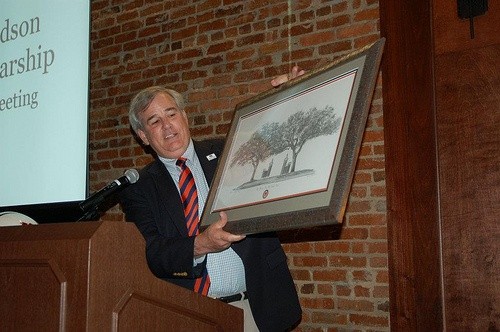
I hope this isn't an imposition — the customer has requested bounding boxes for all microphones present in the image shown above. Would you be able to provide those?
[79,169,140,212]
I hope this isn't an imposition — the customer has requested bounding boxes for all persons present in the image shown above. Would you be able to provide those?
[118,66,306,332]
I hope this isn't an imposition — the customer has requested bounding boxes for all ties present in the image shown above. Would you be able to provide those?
[175,157,211,297]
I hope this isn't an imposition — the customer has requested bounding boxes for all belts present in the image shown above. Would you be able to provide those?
[214,292,249,304]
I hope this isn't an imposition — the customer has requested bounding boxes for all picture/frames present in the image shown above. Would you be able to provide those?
[198,37,386,236]
